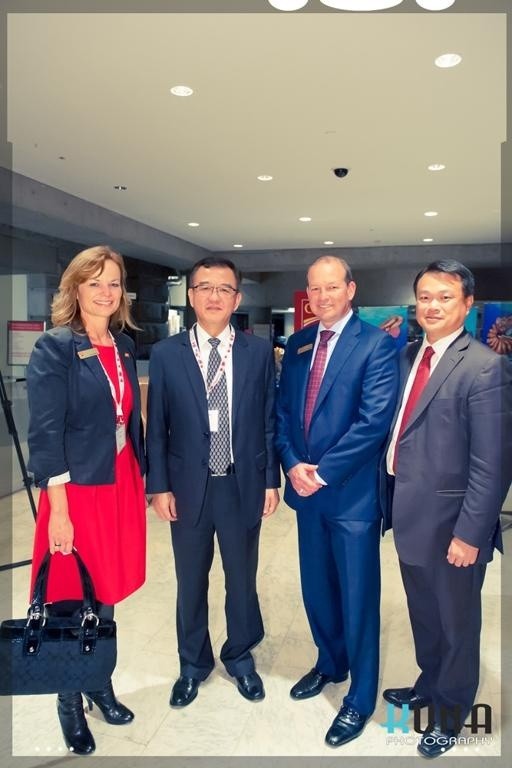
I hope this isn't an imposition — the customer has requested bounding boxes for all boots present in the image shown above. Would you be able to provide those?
[83,601,134,725]
[44,601,95,755]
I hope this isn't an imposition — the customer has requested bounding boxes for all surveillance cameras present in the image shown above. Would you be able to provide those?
[336,168,348,177]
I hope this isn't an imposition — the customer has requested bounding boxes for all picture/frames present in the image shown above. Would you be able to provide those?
[7,320,45,366]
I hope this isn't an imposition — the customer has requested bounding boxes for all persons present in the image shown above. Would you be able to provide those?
[379,259,512,758]
[25,246,148,755]
[146,257,281,706]
[275,255,401,747]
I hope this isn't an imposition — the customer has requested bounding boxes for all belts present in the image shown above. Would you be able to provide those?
[209,463,236,477]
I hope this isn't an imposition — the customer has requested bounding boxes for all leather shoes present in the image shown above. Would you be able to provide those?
[169,666,214,707]
[417,722,458,758]
[383,687,430,711]
[325,703,367,747]
[290,667,348,699]
[236,671,265,701]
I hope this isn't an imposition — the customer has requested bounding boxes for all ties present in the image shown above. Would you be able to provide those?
[303,330,336,438]
[206,337,232,474]
[393,345,436,473]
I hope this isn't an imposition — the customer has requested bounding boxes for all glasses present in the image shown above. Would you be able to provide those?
[192,284,241,297]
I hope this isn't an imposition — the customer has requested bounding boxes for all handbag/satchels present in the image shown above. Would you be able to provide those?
[0,609,118,697]
